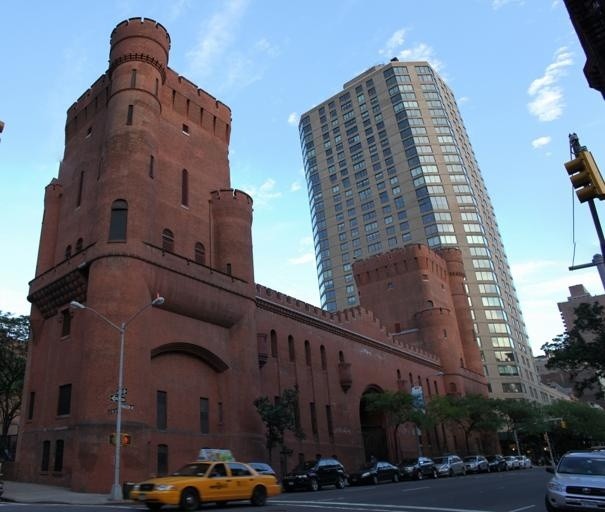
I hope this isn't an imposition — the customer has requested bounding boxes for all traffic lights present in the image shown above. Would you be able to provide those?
[564,153,605,204]
[105,430,133,448]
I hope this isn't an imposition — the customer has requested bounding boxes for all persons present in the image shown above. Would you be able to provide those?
[370,455,376,464]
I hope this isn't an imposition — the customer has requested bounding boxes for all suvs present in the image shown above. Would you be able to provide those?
[544,449,605,512]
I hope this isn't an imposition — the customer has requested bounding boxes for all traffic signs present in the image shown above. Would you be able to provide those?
[104,386,135,417]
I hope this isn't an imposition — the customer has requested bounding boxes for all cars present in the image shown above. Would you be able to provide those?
[400,456,439,480]
[281,455,349,491]
[130,449,281,506]
[243,460,279,484]
[432,455,466,478]
[346,462,401,485]
[464,453,533,473]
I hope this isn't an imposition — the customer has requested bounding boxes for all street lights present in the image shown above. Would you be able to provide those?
[70,295,171,502]
